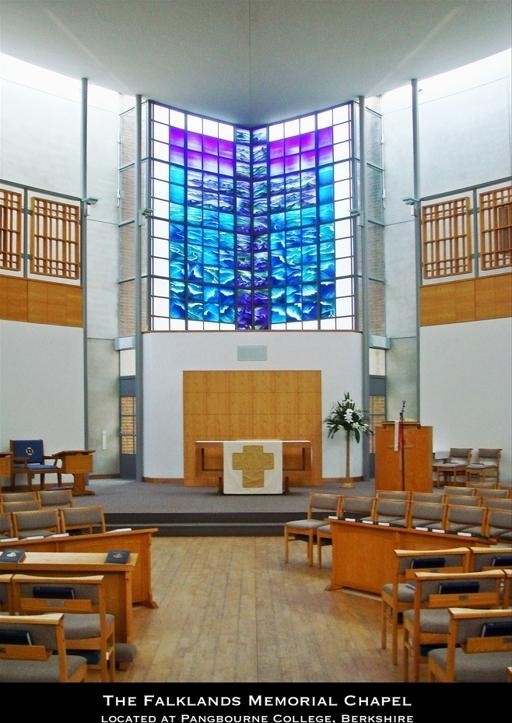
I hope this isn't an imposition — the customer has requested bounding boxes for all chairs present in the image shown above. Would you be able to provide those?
[10,440,62,492]
[436,448,473,488]
[432,452,449,487]
[1,450,159,683]
[462,448,502,489]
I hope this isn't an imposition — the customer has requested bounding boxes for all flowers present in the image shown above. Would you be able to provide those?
[323,392,375,443]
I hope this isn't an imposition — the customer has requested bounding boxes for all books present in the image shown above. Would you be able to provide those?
[0,549,26,564]
[410,556,447,569]
[0,628,33,645]
[490,555,511,566]
[32,585,76,599]
[0,531,70,543]
[437,581,480,594]
[104,549,130,564]
[328,514,489,540]
[105,528,133,533]
[480,621,512,636]
